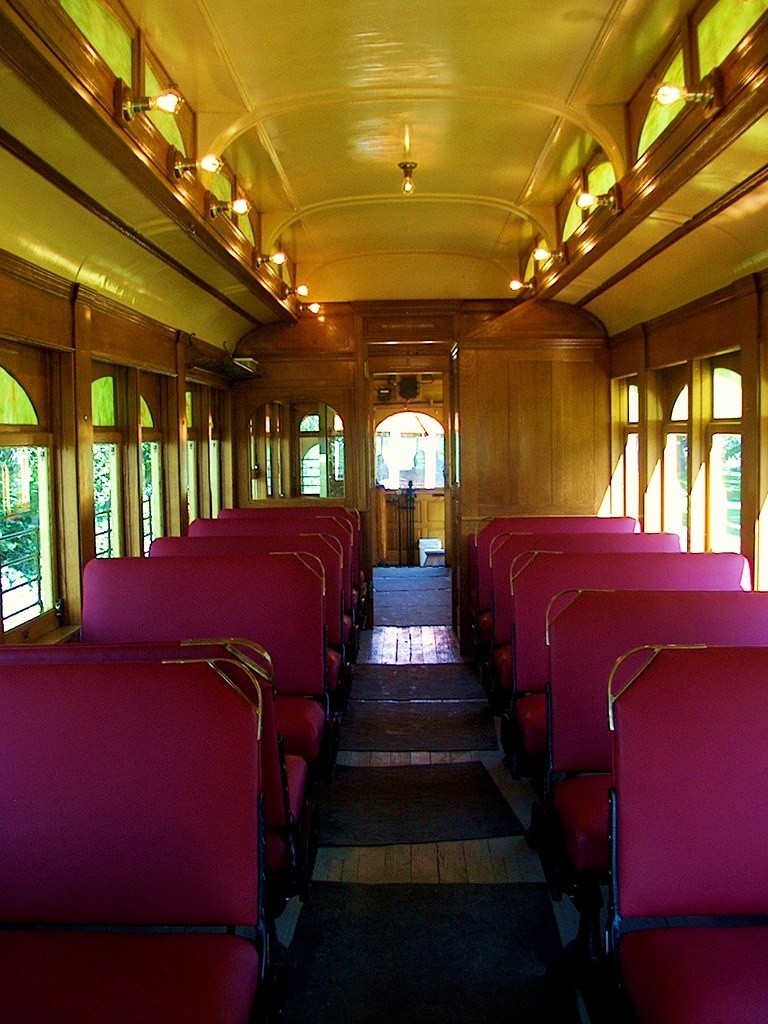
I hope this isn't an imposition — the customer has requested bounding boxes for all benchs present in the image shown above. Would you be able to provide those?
[0,507,768,1024]
